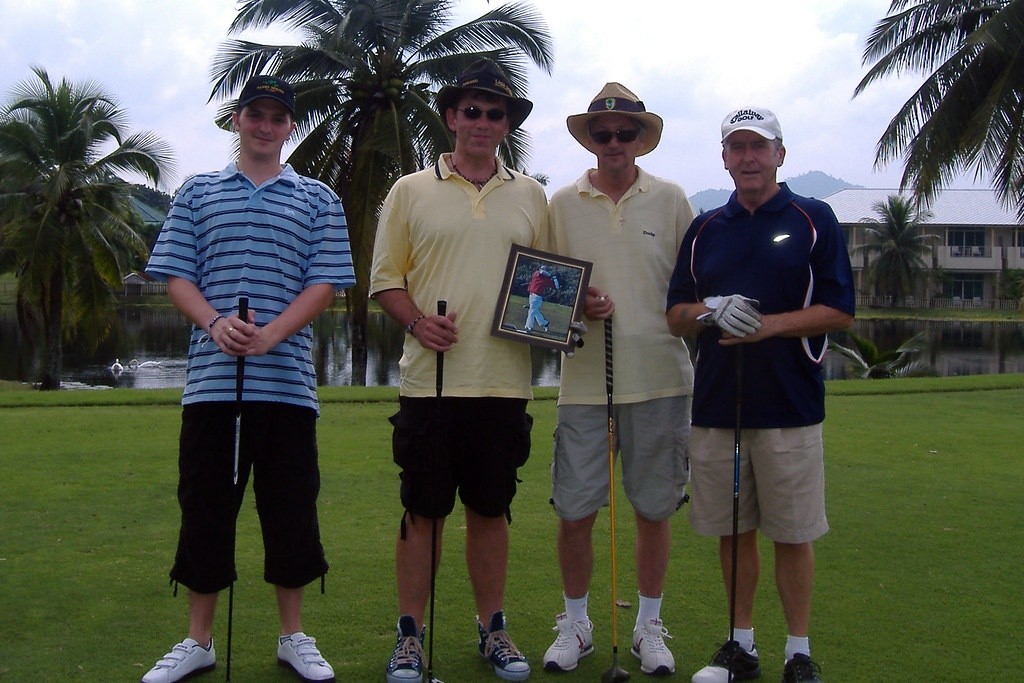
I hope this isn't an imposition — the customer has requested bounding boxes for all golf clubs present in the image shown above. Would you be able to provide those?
[422,300,450,683]
[223,293,250,682]
[601,292,632,682]
[728,340,746,683]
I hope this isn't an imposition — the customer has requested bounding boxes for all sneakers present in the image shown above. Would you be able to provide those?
[385,615,428,683]
[543,612,594,671]
[476,611,530,681]
[691,640,761,683]
[782,653,822,683]
[630,616,675,674]
[278,631,336,683]
[141,638,215,683]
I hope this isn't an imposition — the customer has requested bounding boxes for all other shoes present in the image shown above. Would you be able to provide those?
[543,321,549,332]
[524,326,533,330]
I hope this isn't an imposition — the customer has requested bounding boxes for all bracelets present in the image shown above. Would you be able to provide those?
[208,314,227,339]
[406,314,425,336]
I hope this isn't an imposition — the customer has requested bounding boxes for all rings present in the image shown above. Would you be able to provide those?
[600,296,605,302]
[228,328,236,337]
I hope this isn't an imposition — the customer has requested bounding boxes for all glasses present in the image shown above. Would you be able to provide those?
[453,106,509,120]
[588,127,641,144]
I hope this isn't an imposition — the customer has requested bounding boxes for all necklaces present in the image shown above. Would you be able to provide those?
[449,154,498,189]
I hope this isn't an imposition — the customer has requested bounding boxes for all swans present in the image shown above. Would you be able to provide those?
[129,359,161,369]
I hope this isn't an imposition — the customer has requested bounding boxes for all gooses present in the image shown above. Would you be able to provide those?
[112,358,123,371]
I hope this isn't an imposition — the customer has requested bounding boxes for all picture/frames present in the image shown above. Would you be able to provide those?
[489,244,594,353]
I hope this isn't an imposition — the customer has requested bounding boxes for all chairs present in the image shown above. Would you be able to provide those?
[952,246,961,256]
[973,297,981,308]
[906,295,914,306]
[972,247,981,257]
[953,296,961,307]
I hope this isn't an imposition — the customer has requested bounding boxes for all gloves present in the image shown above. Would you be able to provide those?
[696,294,762,338]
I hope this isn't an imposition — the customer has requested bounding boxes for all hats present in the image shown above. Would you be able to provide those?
[436,59,533,134]
[237,76,295,118]
[721,107,783,147]
[567,83,662,156]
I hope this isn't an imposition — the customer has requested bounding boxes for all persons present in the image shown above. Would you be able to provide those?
[525,265,560,331]
[665,105,856,683]
[369,57,549,683]
[542,80,697,673]
[142,75,357,683]
